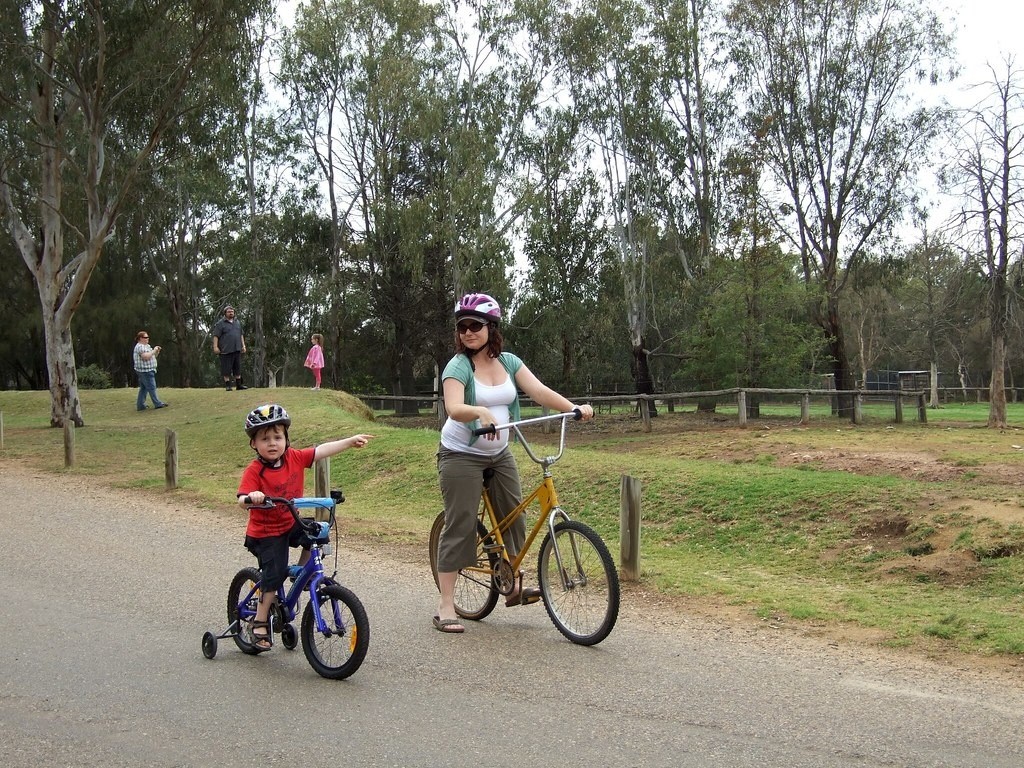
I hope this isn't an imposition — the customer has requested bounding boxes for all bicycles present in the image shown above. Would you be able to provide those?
[428,406,621,646]
[200,490,370,680]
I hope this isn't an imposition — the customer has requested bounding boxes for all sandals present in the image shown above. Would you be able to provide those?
[247,615,272,654]
[290,565,318,591]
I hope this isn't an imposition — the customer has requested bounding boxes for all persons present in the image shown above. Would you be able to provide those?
[237,402,375,651]
[304,334,325,391]
[431,292,592,632]
[133,331,168,411]
[212,307,251,391]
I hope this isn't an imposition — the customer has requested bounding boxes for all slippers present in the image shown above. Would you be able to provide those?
[433,616,465,632]
[505,587,542,607]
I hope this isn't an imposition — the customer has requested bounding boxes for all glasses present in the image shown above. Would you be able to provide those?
[457,321,490,334]
[142,336,150,339]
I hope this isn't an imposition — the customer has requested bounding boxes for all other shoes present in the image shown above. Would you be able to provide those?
[155,403,168,410]
[311,387,320,391]
[236,384,248,390]
[137,406,149,412]
[226,386,232,391]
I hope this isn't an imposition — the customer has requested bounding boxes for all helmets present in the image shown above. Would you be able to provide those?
[245,404,291,438]
[454,293,501,326]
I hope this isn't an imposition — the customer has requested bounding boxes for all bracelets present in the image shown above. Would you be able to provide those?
[572,404,578,411]
[242,346,246,348]
